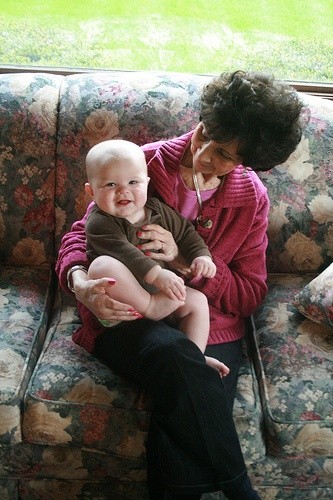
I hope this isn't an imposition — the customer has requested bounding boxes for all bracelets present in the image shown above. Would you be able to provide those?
[66,265,88,292]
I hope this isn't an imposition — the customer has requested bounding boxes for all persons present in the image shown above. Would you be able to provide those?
[56,68,311,500]
[84,139,231,381]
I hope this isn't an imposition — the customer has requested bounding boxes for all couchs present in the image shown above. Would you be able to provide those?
[0,72,332,499]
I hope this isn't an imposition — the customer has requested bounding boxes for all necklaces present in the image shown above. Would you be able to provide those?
[192,168,225,224]
